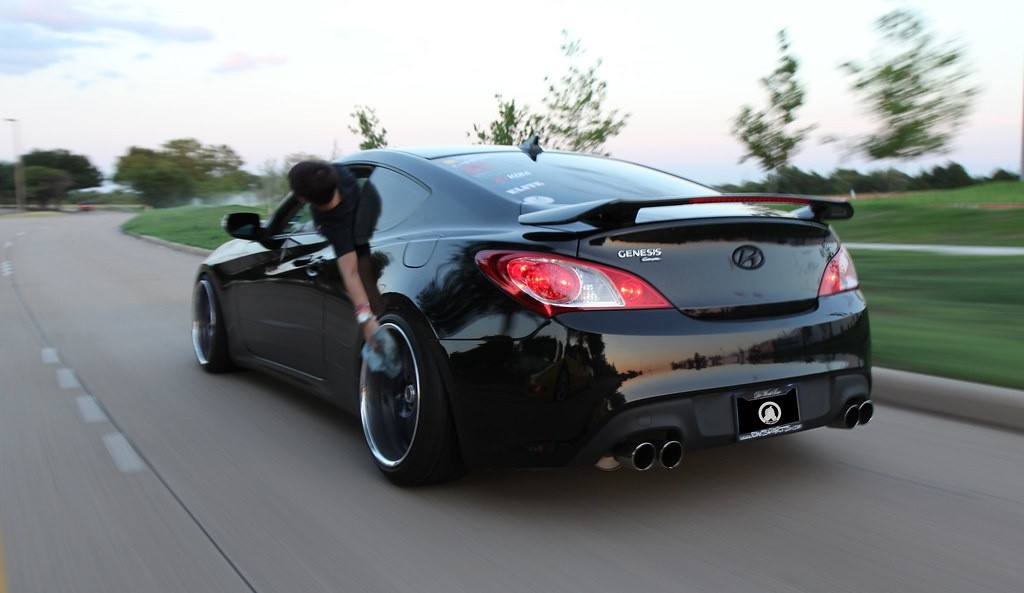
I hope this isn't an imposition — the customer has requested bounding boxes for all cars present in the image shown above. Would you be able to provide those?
[191,134,874,486]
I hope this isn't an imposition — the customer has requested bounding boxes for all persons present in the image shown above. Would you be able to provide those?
[290,159,390,358]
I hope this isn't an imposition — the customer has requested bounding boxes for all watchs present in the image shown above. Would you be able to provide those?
[357,312,374,324]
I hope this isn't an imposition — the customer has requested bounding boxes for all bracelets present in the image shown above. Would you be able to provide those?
[352,304,370,316]
